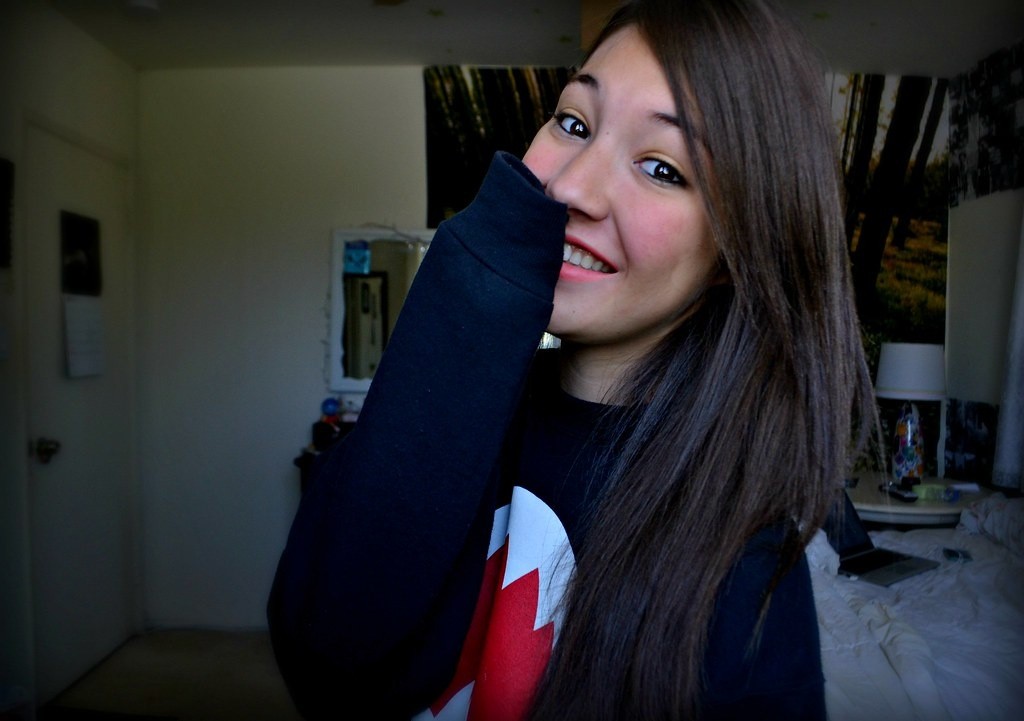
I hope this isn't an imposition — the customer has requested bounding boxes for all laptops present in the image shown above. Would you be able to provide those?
[820,487,941,588]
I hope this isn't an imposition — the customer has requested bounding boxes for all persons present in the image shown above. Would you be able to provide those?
[266,0,888,720]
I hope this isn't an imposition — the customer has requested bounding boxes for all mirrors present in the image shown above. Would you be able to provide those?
[346,238,429,379]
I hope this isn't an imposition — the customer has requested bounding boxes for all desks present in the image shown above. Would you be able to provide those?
[848,476,987,530]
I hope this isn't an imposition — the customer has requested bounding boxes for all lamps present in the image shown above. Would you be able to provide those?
[875,342,949,488]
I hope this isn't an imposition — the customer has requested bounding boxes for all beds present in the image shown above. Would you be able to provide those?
[800,500,1024,721]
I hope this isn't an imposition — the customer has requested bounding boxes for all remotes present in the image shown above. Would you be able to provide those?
[879,483,919,502]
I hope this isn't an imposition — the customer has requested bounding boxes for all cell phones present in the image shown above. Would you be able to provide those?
[944,548,972,561]
